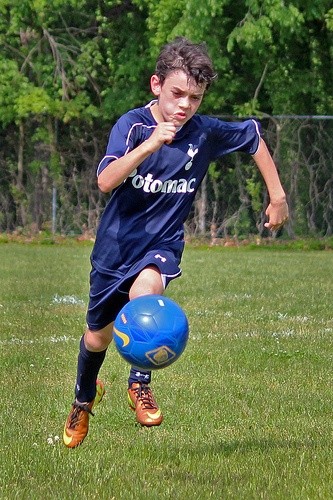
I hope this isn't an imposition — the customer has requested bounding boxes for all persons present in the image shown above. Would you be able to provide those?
[61,43,289,449]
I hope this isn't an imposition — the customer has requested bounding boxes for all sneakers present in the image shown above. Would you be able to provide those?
[126,382,163,427]
[63,380,105,449]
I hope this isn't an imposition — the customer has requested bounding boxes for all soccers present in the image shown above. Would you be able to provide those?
[112,294,189,369]
